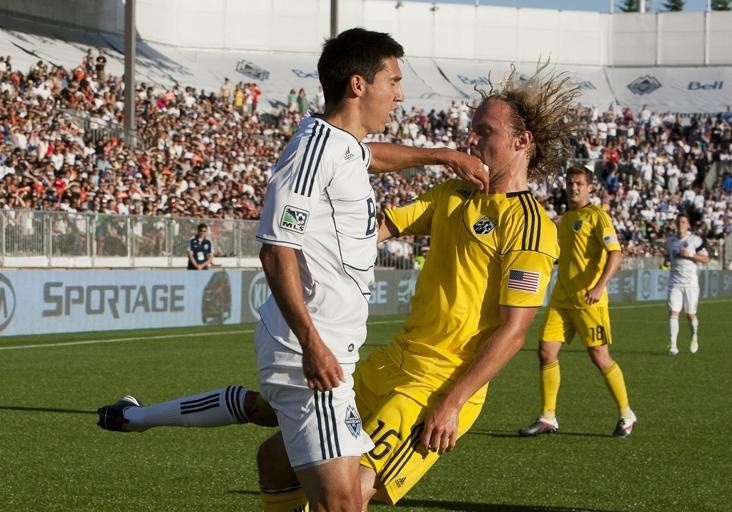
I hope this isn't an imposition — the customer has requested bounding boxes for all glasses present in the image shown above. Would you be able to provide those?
[199,230,207,232]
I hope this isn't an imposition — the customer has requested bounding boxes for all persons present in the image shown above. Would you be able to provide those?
[661,214,709,353]
[518,164,638,438]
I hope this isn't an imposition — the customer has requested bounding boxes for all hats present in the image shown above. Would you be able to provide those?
[0,49,109,77]
[0,78,260,216]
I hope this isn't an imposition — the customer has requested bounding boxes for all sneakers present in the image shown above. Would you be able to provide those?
[97,395,144,432]
[519,416,558,436]
[613,411,637,438]
[667,348,678,355]
[689,335,698,353]
